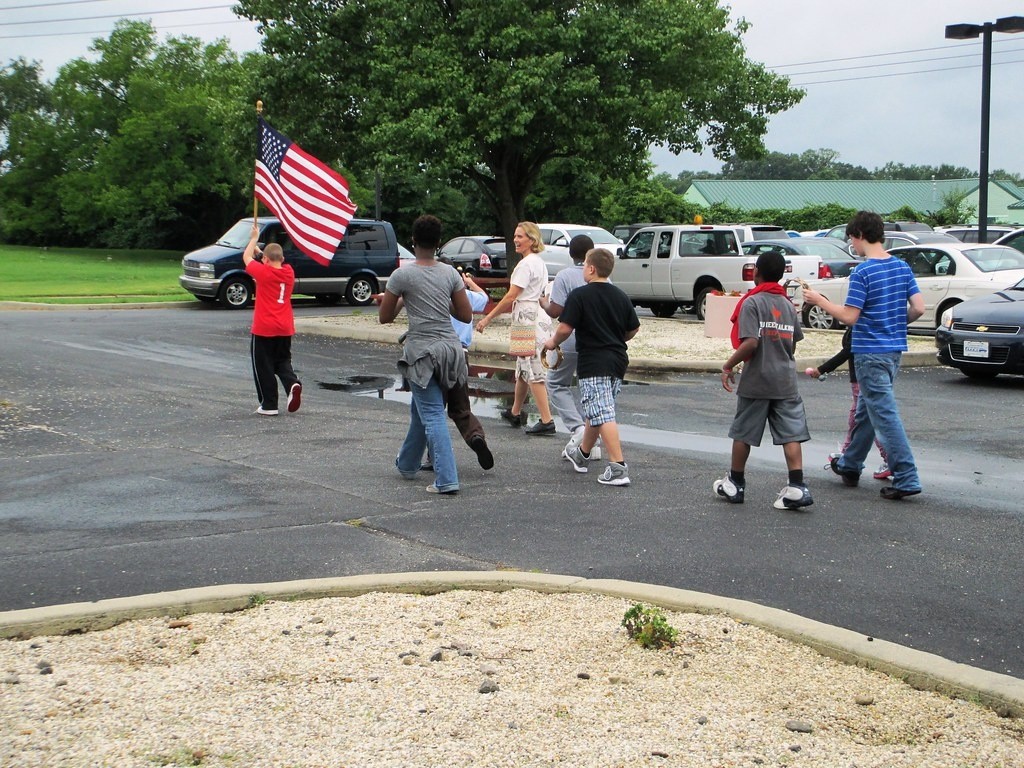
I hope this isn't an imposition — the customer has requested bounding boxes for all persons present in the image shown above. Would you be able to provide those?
[538,234,613,461]
[805,326,892,479]
[713,251,814,510]
[243,226,302,415]
[544,249,640,486]
[370,257,494,471]
[379,216,473,494]
[475,222,558,434]
[802,211,926,500]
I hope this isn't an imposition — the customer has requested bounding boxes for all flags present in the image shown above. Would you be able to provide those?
[254,114,358,266]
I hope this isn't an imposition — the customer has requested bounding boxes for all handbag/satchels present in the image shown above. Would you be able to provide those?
[508,326,536,356]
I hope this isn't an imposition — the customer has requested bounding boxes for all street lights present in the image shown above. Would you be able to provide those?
[945,16,1024,243]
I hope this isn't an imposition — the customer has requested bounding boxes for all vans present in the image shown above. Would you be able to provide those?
[179,216,401,309]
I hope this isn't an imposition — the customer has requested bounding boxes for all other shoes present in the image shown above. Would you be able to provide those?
[397,452,400,458]
[420,463,434,470]
[426,485,459,493]
[471,436,494,470]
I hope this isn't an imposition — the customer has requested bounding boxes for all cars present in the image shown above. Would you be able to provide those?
[399,242,416,274]
[533,223,1024,383]
[435,237,507,277]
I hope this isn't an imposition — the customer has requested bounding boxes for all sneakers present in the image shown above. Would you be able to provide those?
[831,457,860,487]
[873,464,891,479]
[880,487,922,499]
[713,471,747,504]
[500,409,522,428]
[287,383,302,412]
[565,445,591,472]
[828,453,844,462]
[256,406,278,416]
[588,446,602,460]
[524,419,557,436]
[561,424,585,460]
[597,461,630,485]
[773,479,814,510]
